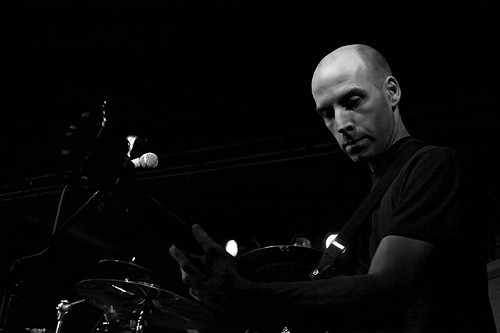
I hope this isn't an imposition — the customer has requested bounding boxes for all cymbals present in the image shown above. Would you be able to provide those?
[75,278,215,331]
[97,258,154,281]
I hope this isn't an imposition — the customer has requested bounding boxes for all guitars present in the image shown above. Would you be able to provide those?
[1,96,110,327]
[65,99,374,283]
[1,150,160,202]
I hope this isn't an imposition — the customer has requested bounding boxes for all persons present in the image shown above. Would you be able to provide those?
[168,43,497,333]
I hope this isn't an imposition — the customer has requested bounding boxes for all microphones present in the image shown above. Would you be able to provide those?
[80,152,159,182]
